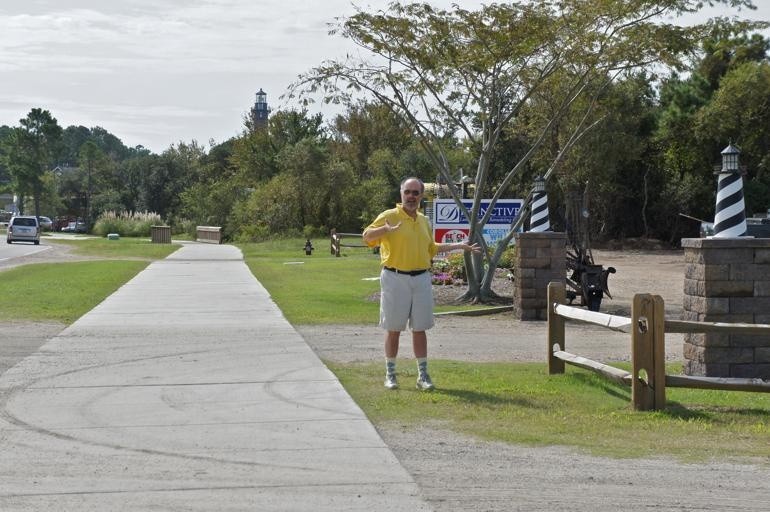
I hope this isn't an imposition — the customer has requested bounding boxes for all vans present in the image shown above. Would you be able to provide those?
[4,216,40,244]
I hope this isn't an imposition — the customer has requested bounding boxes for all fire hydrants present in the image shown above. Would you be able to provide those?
[301,239,315,256]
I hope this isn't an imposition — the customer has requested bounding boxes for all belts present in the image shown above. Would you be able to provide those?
[384,266,426,276]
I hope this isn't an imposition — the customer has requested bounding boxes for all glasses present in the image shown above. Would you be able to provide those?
[404,190,419,195]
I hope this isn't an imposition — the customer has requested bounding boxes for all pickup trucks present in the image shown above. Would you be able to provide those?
[699,220,770,239]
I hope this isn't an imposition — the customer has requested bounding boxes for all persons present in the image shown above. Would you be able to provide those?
[362,176,483,393]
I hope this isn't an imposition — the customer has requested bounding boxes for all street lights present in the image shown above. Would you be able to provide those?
[461,175,471,198]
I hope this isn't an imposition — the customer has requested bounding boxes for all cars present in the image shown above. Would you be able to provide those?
[0,213,85,233]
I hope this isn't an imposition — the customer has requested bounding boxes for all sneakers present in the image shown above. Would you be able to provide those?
[385,374,398,388]
[416,375,433,390]
